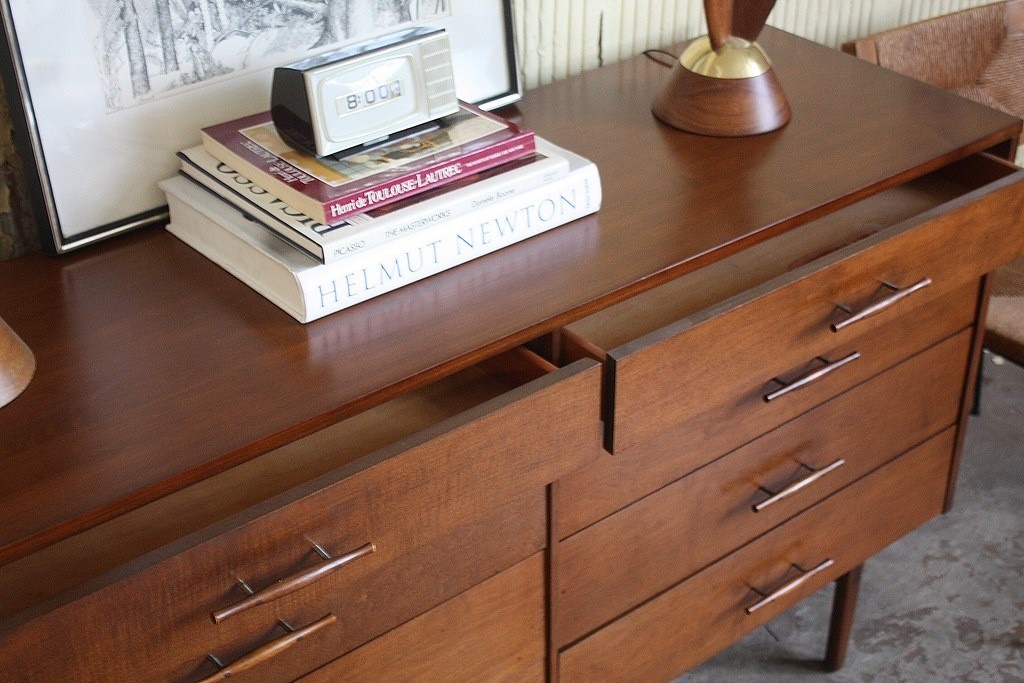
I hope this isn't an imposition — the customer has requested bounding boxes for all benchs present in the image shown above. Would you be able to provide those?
[842,0,1024,415]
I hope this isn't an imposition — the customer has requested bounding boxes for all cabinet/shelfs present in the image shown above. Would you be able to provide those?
[0,22,1023,683]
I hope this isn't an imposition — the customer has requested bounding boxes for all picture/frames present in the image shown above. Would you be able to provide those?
[0,0,523,253]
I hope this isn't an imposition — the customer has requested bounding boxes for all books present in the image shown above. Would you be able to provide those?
[152,99,602,324]
